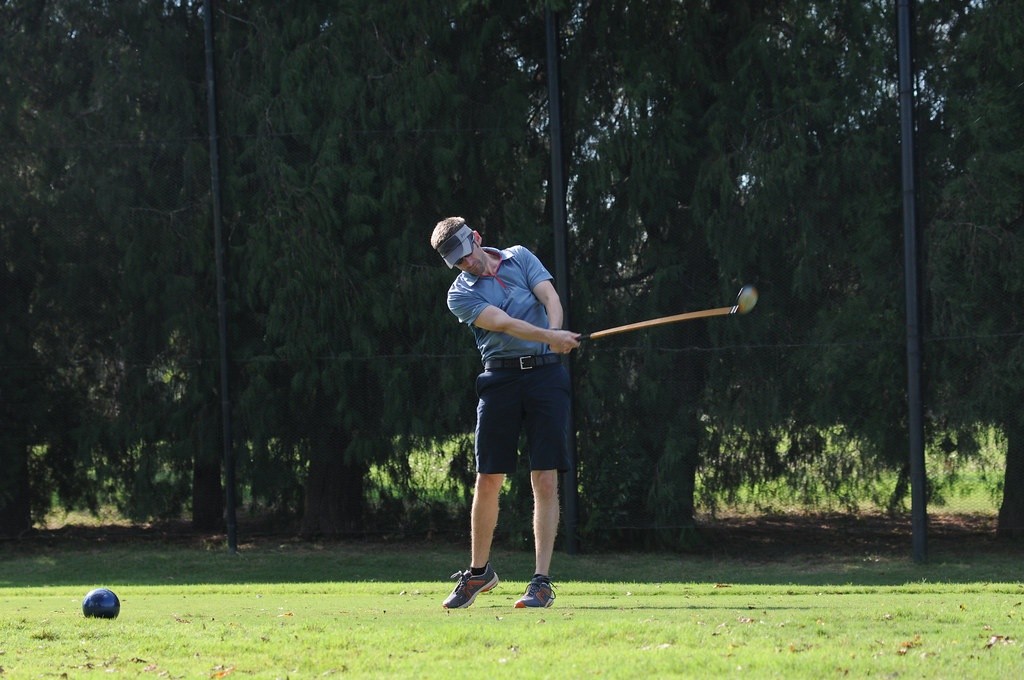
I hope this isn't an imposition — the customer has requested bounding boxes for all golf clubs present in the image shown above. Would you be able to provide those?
[573,283,760,341]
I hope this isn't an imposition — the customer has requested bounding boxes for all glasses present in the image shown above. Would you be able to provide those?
[456,236,475,264]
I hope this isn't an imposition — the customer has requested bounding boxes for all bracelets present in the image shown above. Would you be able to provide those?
[553,329,560,330]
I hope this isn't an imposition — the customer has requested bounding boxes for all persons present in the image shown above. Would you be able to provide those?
[431,217,581,612]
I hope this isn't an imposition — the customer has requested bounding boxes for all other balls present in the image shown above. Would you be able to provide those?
[84,588,121,620]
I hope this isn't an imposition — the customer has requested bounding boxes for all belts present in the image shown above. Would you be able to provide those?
[485,354,561,370]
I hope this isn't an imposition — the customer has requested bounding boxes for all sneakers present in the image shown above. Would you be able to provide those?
[515,577,557,608]
[443,562,499,609]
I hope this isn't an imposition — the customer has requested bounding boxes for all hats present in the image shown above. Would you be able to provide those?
[437,224,474,270]
